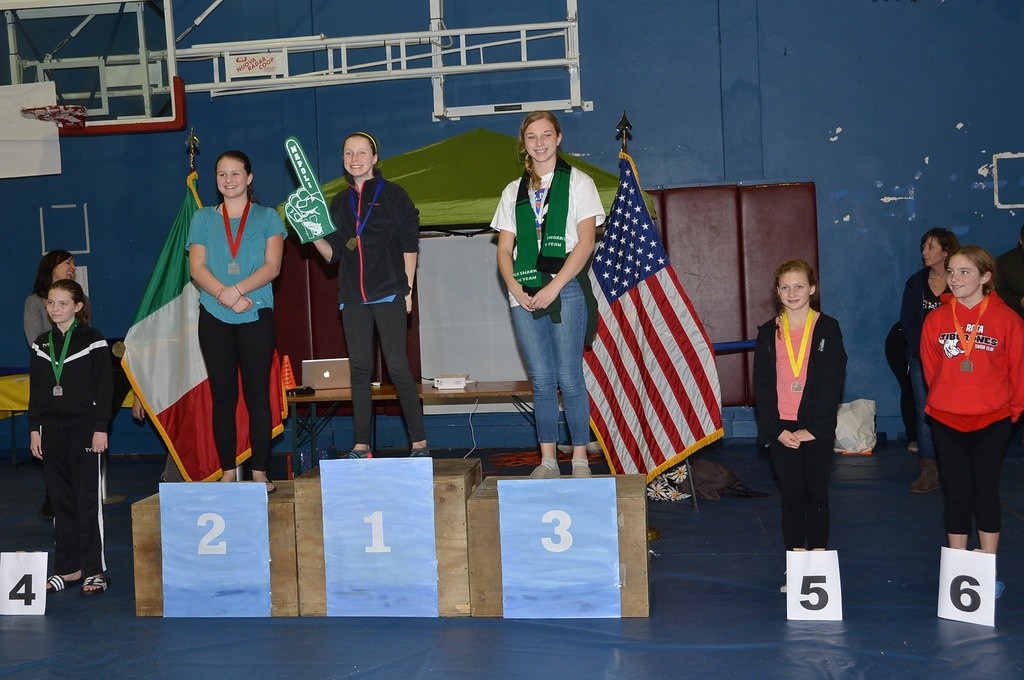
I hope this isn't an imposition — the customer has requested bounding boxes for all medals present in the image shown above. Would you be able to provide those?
[346,237,357,252]
[227,260,240,274]
[53,382,63,396]
[790,379,802,392]
[960,358,973,371]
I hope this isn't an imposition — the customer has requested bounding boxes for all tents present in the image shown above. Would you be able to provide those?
[279,125,700,505]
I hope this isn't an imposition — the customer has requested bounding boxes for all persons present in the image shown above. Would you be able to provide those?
[752,259,848,594]
[886,322,919,455]
[921,244,1024,600]
[22,250,90,347]
[29,279,114,595]
[902,228,959,496]
[312,132,431,459]
[131,393,183,482]
[184,149,290,494]
[994,226,1024,319]
[488,111,606,477]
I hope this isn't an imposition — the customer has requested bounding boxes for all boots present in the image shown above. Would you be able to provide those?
[908,459,941,492]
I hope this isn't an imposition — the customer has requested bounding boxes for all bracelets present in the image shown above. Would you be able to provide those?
[234,284,244,297]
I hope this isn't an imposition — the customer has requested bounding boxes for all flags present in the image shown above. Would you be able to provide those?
[582,149,725,484]
[119,171,288,482]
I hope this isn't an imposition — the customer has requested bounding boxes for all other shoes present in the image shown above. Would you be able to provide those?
[410,448,430,457]
[344,449,372,458]
[995,580,1004,599]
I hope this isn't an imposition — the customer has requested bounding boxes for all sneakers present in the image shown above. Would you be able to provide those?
[571,461,592,478]
[527,465,561,479]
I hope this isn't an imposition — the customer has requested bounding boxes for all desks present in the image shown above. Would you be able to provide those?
[282,376,565,478]
[0,369,141,470]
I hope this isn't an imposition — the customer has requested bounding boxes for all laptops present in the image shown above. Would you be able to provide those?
[301,358,351,389]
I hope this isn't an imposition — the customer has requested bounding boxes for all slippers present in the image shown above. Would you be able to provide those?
[253,476,277,493]
[47,574,83,593]
[81,577,111,595]
